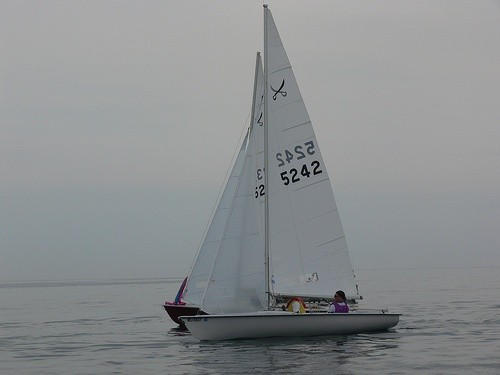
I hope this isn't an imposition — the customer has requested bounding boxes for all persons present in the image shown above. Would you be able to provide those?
[287,296,306,313]
[328,291,350,313]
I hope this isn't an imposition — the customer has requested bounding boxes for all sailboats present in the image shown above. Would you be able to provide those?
[162,51,264,326]
[179,4,403,341]
